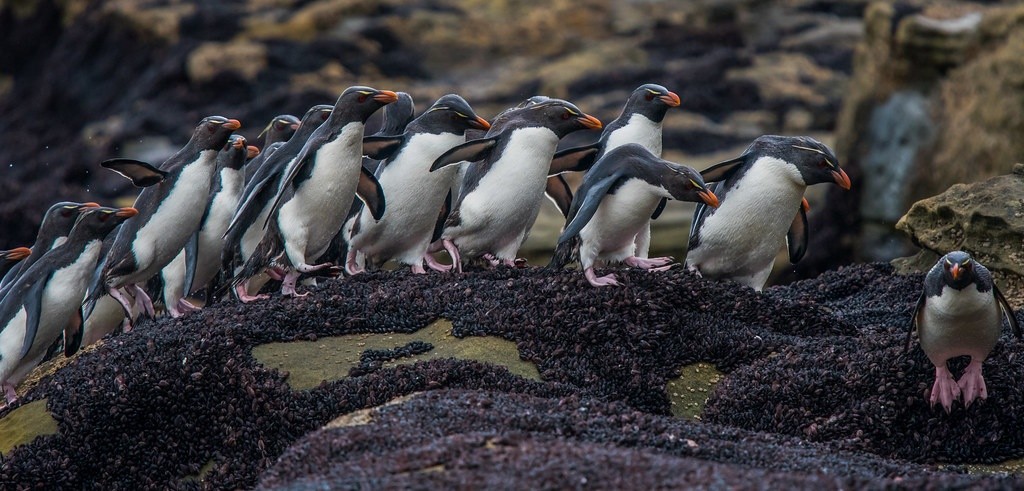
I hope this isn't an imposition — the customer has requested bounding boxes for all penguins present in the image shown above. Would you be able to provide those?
[0,84,850,410]
[904,251,1021,415]
